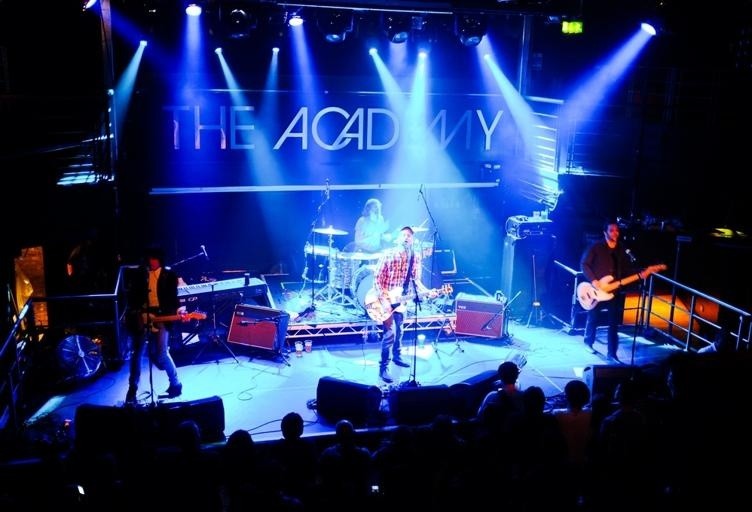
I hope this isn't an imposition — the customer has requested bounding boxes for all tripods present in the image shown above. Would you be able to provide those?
[265,320,291,368]
[526,255,556,330]
[407,227,442,313]
[291,218,342,324]
[191,286,240,363]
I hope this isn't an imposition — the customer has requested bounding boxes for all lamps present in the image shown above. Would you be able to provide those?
[83,0,670,42]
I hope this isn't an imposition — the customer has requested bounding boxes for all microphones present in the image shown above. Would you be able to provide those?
[200,244,208,258]
[325,178,330,200]
[417,183,423,200]
[625,248,637,261]
[405,243,416,256]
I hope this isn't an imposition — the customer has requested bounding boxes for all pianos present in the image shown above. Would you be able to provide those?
[178,278,267,307]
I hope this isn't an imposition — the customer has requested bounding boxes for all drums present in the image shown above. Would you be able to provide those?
[302,245,338,284]
[351,266,377,309]
[330,251,372,288]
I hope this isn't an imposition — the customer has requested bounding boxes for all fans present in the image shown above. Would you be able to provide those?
[57,335,104,380]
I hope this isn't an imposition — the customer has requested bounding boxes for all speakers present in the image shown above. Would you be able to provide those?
[500,233,555,315]
[666,343,732,401]
[388,385,450,424]
[227,304,290,353]
[582,363,640,411]
[74,403,153,443]
[157,396,226,443]
[316,376,382,427]
[450,369,498,415]
[454,292,506,339]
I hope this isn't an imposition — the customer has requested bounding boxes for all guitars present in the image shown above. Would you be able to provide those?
[577,264,667,310]
[365,284,454,322]
[126,313,206,336]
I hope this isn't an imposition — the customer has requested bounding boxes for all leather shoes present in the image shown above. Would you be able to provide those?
[586,342,620,364]
[380,366,392,382]
[159,388,180,398]
[395,360,410,367]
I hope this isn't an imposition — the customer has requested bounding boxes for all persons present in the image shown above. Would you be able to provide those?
[373,225,438,383]
[124,248,189,406]
[354,198,392,253]
[582,219,650,367]
[1,347,733,511]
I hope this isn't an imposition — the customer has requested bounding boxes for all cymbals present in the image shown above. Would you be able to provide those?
[312,229,348,235]
[410,227,429,232]
